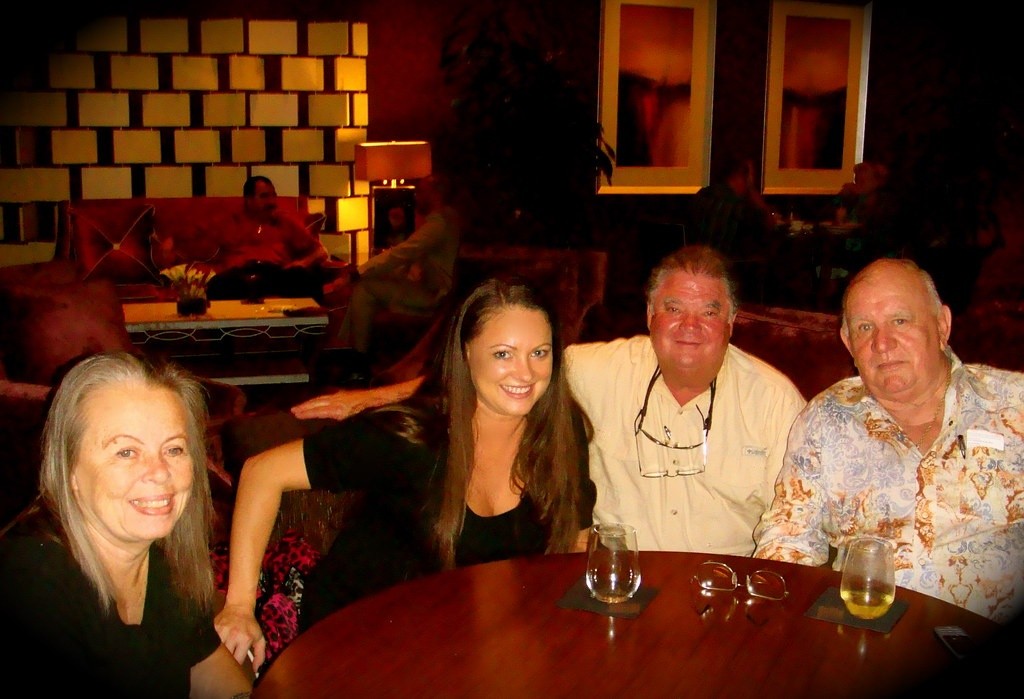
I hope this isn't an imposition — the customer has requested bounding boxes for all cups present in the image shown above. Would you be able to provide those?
[790,221,803,232]
[839,539,895,620]
[834,208,847,226]
[587,524,640,603]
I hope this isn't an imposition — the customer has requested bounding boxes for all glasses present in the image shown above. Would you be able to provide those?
[637,426,707,450]
[635,405,708,478]
[689,562,787,627]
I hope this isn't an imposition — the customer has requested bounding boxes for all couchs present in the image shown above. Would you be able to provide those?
[53,195,342,299]
[729,298,861,404]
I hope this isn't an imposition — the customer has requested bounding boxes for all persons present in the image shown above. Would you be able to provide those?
[815,163,892,301]
[752,257,1023,635]
[0,350,253,699]
[323,171,460,387]
[962,185,1024,318]
[194,176,329,300]
[291,245,807,558]
[214,275,598,687]
[669,150,781,306]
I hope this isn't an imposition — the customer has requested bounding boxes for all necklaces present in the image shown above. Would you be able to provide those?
[916,359,951,450]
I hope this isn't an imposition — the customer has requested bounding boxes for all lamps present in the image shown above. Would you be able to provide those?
[356,138,433,250]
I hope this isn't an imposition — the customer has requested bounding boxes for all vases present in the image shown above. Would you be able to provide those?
[178,295,205,317]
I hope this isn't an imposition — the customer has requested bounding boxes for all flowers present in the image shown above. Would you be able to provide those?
[160,263,216,300]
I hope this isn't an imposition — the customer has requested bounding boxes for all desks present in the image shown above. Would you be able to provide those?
[122,296,328,385]
[250,550,1024,699]
